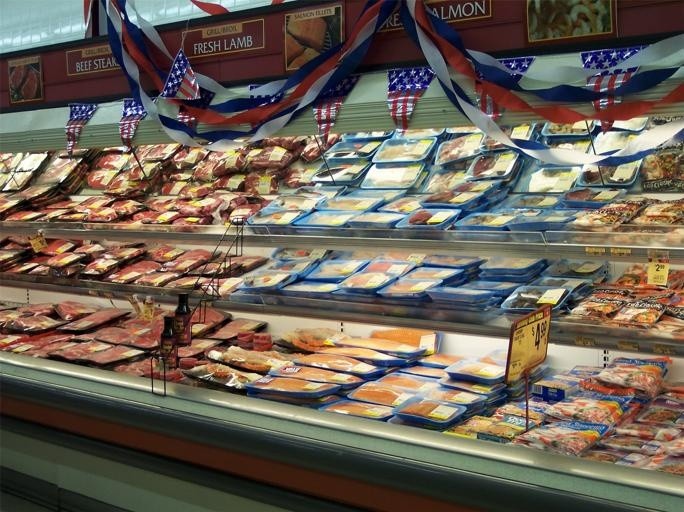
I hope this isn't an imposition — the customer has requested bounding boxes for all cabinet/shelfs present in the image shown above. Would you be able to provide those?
[0,50,684,512]
